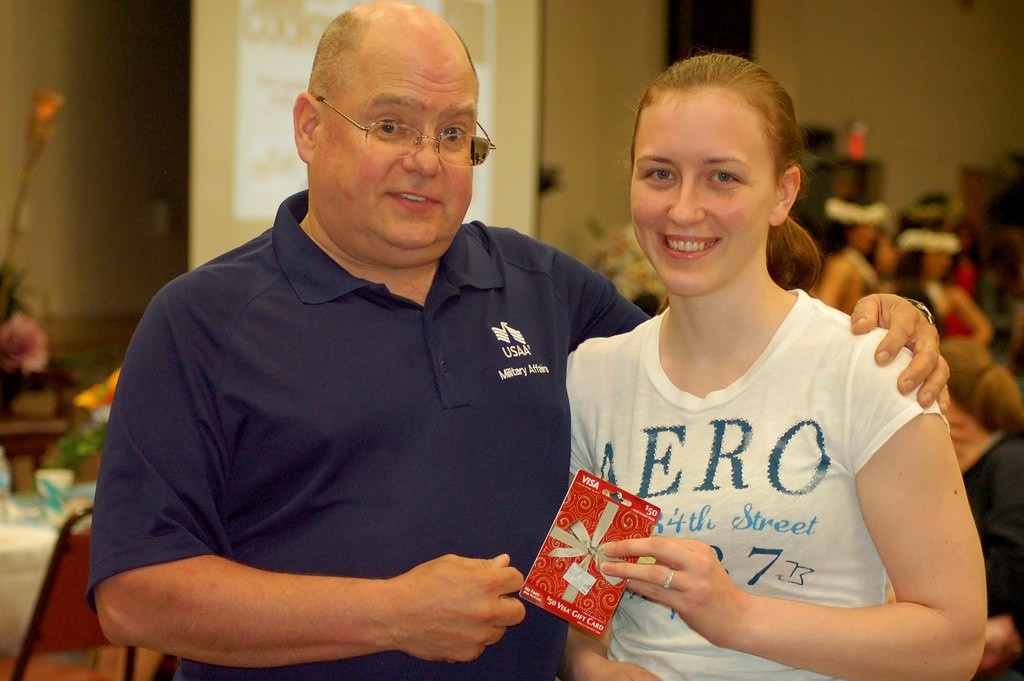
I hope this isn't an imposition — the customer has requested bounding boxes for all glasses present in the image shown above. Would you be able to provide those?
[316,94,496,168]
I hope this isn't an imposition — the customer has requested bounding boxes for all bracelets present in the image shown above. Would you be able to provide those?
[908,298,936,326]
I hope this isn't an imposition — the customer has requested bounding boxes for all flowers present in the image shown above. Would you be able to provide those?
[47,366,120,472]
[0,90,64,418]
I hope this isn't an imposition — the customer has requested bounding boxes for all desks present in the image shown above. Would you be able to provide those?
[1,492,164,680]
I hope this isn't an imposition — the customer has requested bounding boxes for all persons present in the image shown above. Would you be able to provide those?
[565,53,989,681]
[85,0,950,681]
[819,195,1023,680]
[0,272,49,414]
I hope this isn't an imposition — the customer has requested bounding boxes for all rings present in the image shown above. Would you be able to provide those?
[664,567,675,589]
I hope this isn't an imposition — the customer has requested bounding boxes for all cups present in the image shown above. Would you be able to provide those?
[846,121,868,158]
[34,468,75,498]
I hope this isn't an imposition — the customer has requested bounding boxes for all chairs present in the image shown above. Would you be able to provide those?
[9,505,134,681]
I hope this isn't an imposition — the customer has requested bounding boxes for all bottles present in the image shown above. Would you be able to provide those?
[0,447,15,523]
